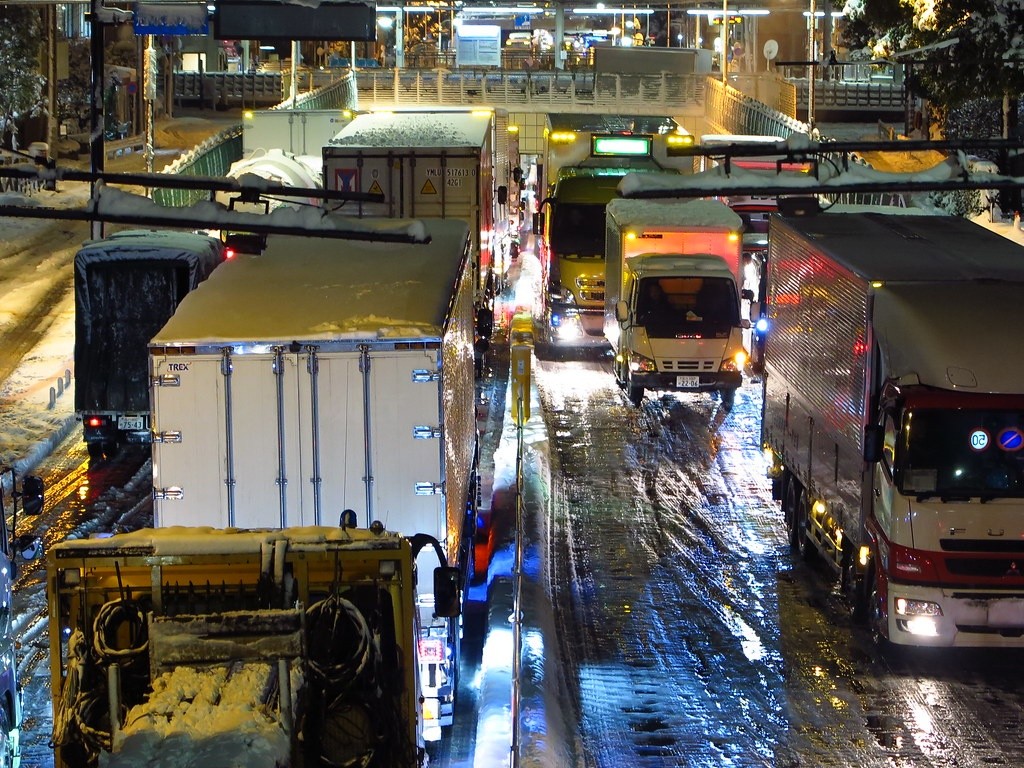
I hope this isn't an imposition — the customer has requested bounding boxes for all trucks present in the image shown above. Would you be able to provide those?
[321,112,526,336]
[147,217,494,741]
[602,198,750,404]
[700,135,817,276]
[0,464,44,768]
[533,113,695,348]
[74,230,228,459]
[760,212,1023,647]
[47,509,460,768]
